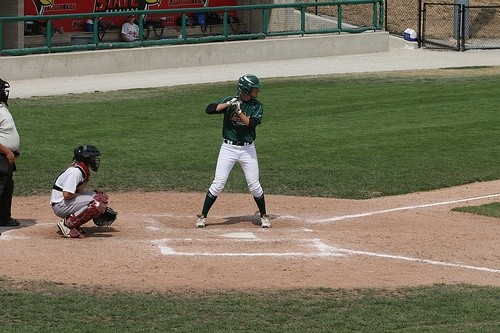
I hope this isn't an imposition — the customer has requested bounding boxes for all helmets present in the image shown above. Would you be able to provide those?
[74,145,100,172]
[0,78,10,105]
[238,75,261,94]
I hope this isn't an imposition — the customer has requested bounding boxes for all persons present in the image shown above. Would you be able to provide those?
[121,15,139,42]
[195,74,271,228]
[0,79,20,227]
[50,144,109,238]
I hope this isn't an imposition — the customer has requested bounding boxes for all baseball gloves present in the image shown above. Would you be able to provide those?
[92,206,118,226]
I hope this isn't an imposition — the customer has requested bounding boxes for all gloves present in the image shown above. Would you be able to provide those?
[227,97,242,116]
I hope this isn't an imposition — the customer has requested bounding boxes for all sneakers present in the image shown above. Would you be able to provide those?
[57,219,86,239]
[259,214,272,228]
[195,214,206,228]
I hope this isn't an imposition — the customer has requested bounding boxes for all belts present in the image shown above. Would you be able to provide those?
[224,139,252,146]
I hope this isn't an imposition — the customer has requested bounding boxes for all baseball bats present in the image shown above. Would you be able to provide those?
[231,92,240,111]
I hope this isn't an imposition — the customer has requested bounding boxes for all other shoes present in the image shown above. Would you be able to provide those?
[0,217,20,226]
